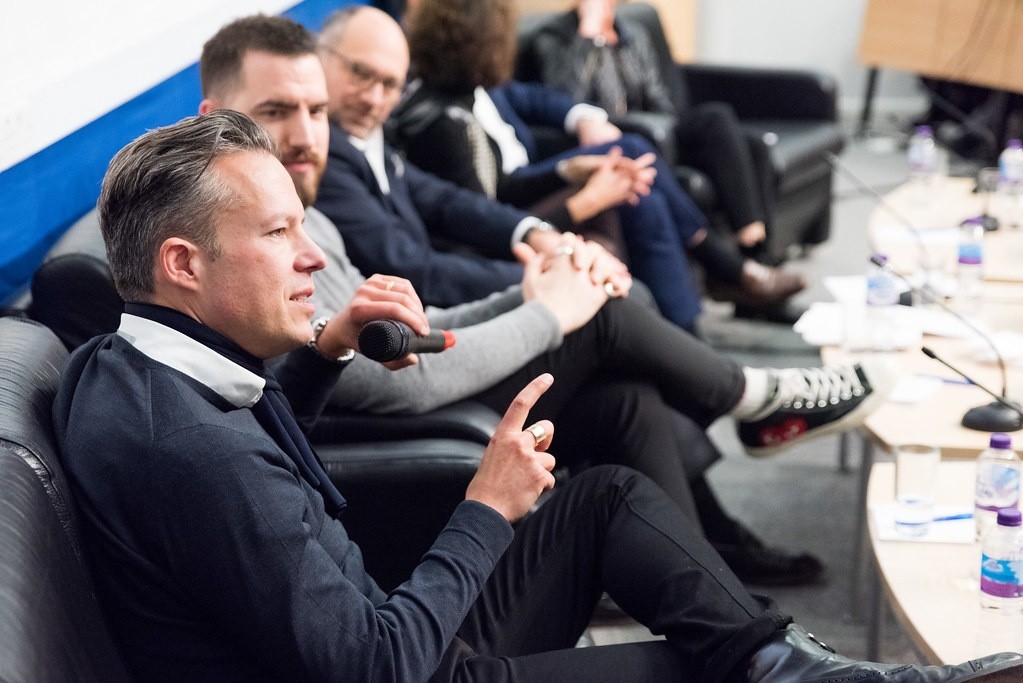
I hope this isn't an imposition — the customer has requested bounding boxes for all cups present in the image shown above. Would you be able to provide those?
[895,444,942,538]
[978,166,1001,231]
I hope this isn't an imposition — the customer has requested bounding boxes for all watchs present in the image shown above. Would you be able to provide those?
[307,317,355,361]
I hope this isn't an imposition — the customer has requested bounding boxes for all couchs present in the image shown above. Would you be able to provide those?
[675,62,846,265]
[0,309,597,683]
[29,206,503,447]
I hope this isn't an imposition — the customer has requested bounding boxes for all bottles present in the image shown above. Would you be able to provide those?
[865,256,896,352]
[998,138,1023,230]
[908,125,938,204]
[981,507,1023,627]
[955,217,984,307]
[974,432,1022,554]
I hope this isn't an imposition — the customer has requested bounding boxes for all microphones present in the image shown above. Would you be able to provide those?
[822,123,1023,432]
[357,317,457,363]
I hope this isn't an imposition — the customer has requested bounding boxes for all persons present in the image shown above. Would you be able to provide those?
[53,108,1022,682]
[312,5,824,581]
[389,0,813,347]
[199,16,884,613]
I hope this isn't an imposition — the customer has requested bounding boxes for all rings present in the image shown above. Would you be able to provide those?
[525,424,546,445]
[386,281,395,292]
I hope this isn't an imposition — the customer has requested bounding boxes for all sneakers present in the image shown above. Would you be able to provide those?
[736,354,896,457]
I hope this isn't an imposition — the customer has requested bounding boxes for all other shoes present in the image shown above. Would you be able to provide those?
[704,261,810,304]
[715,517,823,589]
[736,297,806,324]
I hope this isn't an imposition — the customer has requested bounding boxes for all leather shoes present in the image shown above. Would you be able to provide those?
[740,620,1023,683]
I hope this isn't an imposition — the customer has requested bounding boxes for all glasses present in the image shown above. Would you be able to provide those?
[320,43,405,100]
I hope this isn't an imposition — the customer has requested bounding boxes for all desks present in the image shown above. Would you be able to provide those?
[822,344,1023,624]
[862,459,1023,670]
[798,278,1023,473]
[865,172,1023,281]
[854,0,1023,140]
